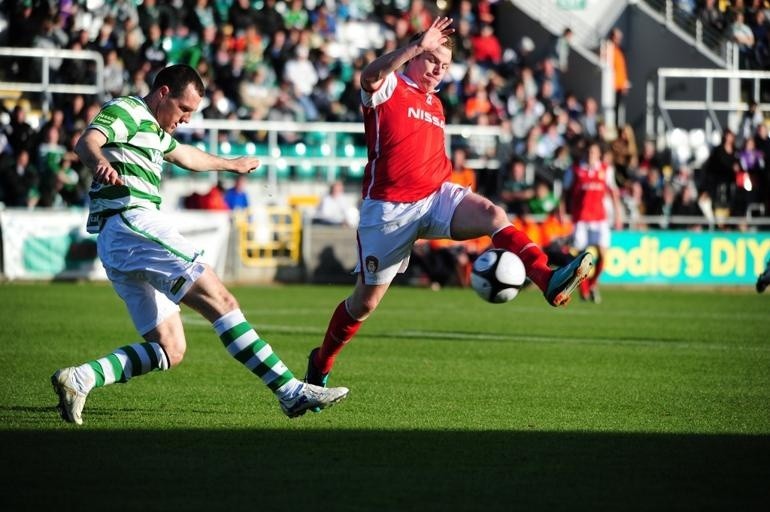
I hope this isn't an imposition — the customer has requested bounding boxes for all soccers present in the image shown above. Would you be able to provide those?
[471,248,526,304]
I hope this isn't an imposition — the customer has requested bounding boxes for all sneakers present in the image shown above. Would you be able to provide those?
[545,249,593,310]
[278,380,350,419]
[303,346,332,414]
[51,365,87,425]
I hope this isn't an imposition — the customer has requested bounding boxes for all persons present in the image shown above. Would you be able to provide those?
[50,64,349,426]
[301,14,594,413]
